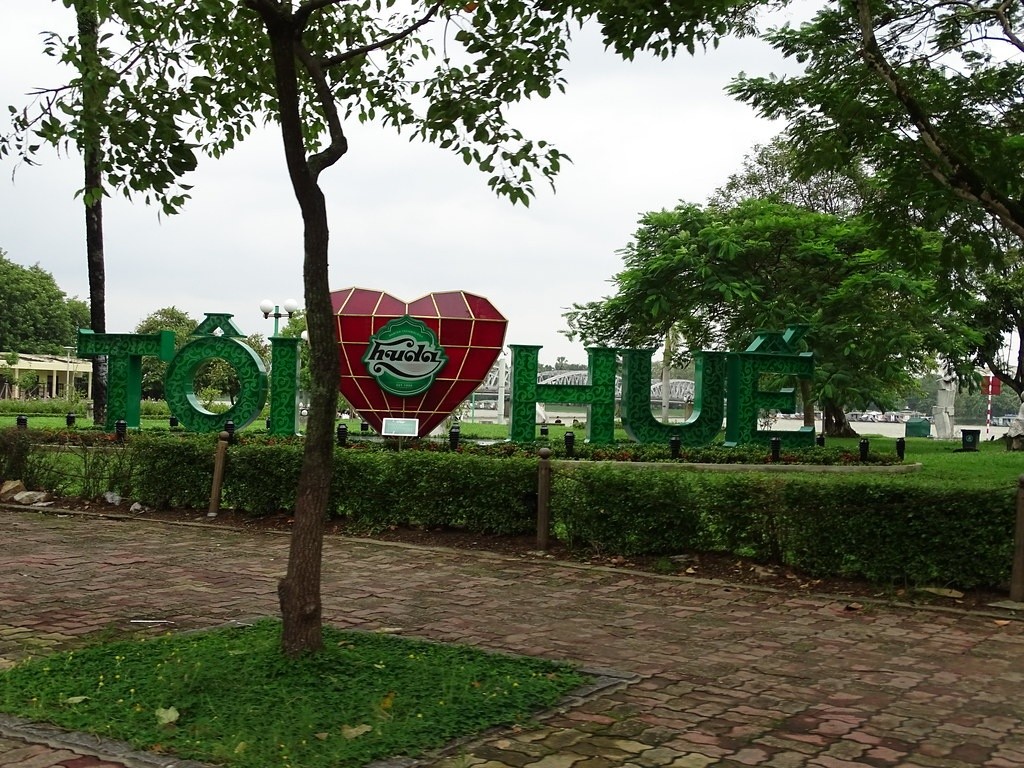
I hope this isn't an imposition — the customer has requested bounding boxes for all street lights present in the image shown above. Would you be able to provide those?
[259,299,298,336]
[63,346,75,402]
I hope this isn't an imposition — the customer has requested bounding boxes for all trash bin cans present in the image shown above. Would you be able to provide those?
[905,417,931,437]
[961,429,981,450]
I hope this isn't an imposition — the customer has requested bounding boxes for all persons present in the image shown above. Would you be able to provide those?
[573,417,578,423]
[555,416,561,424]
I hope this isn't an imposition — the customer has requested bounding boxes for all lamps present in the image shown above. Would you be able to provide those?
[337,423,349,441]
[671,434,681,452]
[361,422,369,430]
[816,434,826,445]
[225,419,235,435]
[16,413,29,427]
[66,410,75,425]
[859,437,870,452]
[266,416,271,429]
[449,421,461,442]
[169,414,179,427]
[541,425,549,435]
[770,436,781,451]
[114,418,127,436]
[896,438,905,451]
[564,430,574,445]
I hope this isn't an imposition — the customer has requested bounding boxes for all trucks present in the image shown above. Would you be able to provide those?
[769,409,825,421]
[845,410,935,424]
[990,417,1016,426]
[459,399,497,410]
